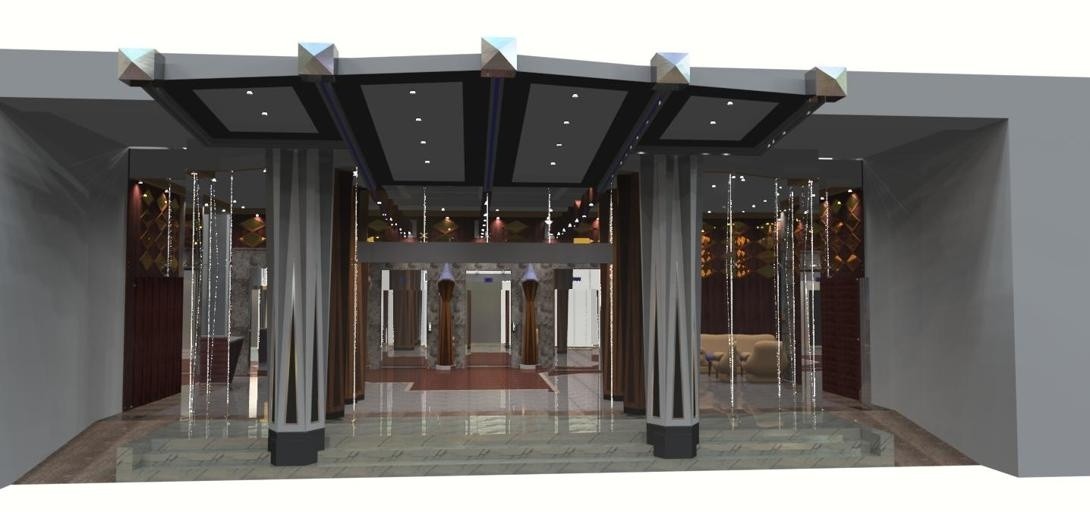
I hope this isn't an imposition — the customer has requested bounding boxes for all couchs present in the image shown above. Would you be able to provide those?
[700,333,777,373]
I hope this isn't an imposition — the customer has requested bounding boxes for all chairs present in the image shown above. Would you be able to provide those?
[740,340,783,383]
[706,352,740,378]
